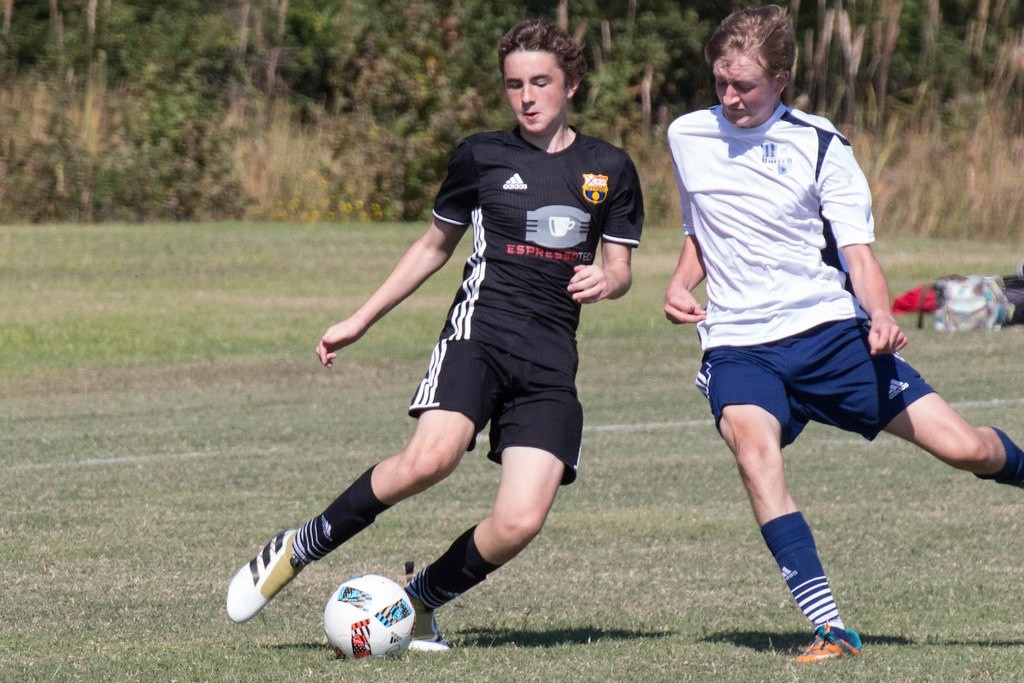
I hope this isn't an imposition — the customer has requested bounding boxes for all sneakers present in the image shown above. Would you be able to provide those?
[403,583,453,652]
[226,528,306,624]
[793,618,863,665]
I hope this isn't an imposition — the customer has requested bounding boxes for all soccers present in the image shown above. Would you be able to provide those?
[322,574,416,661]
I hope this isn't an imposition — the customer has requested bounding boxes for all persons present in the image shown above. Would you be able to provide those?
[664,5,1024,664]
[226,24,646,657]
